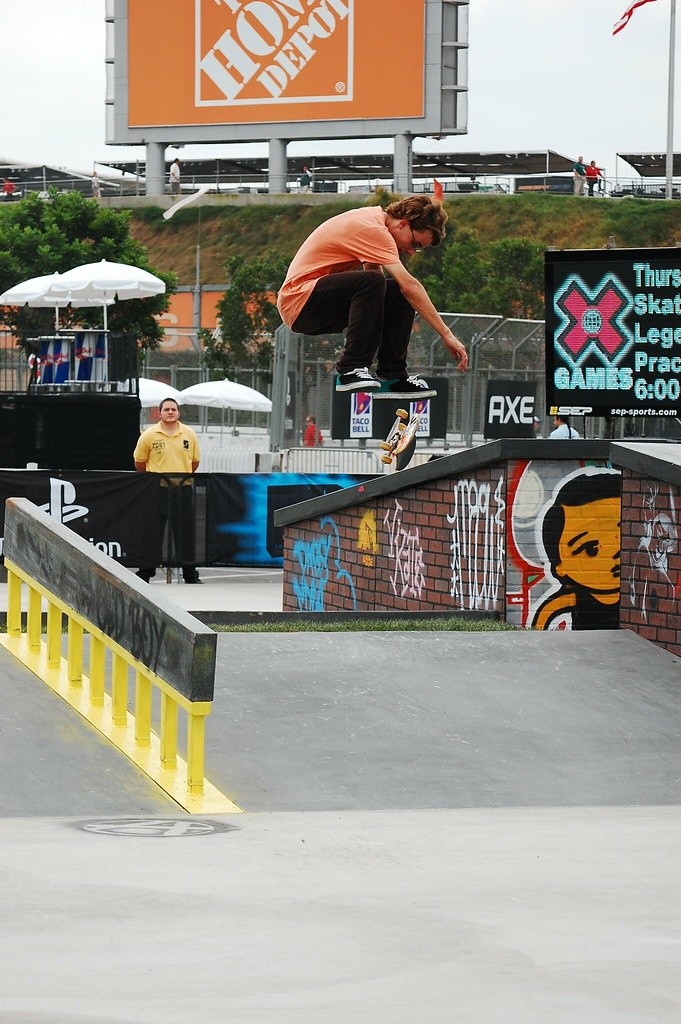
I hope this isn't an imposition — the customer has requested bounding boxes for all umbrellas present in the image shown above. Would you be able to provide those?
[181,378,273,449]
[0,271,116,337]
[48,259,166,380]
[139,377,182,430]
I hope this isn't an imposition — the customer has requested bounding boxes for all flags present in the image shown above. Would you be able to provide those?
[612,0,656,36]
[434,178,444,203]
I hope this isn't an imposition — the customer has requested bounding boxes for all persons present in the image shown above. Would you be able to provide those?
[549,415,580,439]
[169,157,182,195]
[277,197,468,395]
[572,156,587,196]
[301,415,323,447]
[532,415,544,439]
[2,176,17,197]
[91,172,100,197]
[586,160,604,195]
[296,166,313,188]
[133,398,203,584]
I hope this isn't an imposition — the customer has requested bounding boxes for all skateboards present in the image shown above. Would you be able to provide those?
[380,408,420,465]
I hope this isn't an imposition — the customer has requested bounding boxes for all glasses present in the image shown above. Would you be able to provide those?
[408,221,423,254]
[306,420,312,423]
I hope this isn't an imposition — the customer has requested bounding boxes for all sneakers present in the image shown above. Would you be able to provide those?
[336,366,382,393]
[374,374,437,400]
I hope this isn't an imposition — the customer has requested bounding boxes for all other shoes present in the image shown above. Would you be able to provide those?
[135,570,150,583]
[184,576,204,584]
